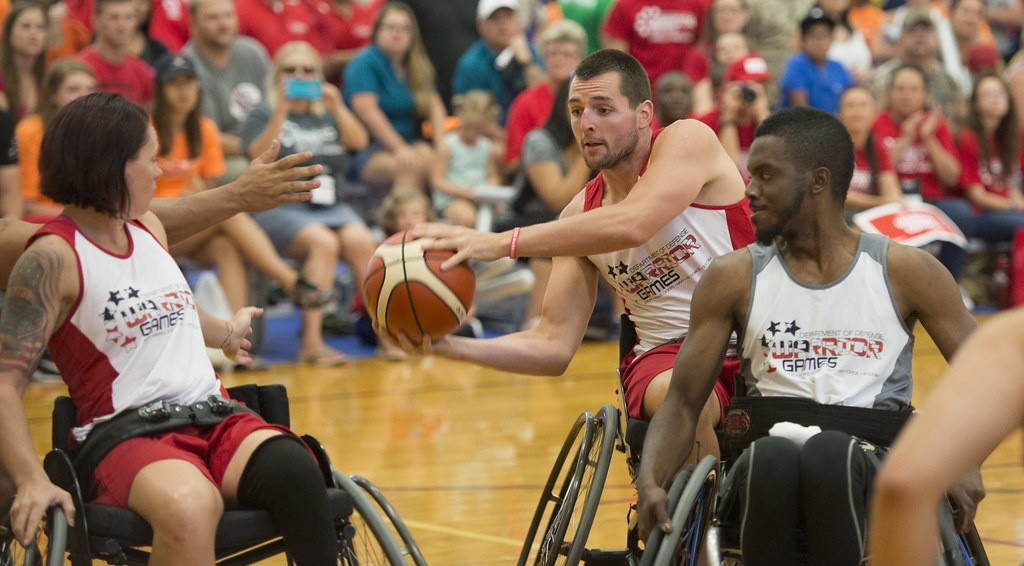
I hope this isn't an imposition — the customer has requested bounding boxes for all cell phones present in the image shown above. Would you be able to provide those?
[285,79,321,100]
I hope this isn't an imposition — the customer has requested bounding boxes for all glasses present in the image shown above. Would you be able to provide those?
[279,65,315,76]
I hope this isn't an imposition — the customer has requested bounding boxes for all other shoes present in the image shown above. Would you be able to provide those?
[298,346,349,367]
[290,276,342,308]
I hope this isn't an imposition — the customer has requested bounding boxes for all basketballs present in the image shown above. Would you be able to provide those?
[361,225,477,344]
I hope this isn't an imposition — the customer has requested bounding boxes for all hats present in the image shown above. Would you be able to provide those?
[154,55,195,78]
[798,9,837,33]
[478,1,519,20]
[903,11,932,31]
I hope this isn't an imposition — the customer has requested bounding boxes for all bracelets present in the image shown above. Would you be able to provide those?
[510,226,520,259]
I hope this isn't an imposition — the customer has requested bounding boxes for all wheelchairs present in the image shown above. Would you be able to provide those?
[0,385,428,566]
[514,314,991,566]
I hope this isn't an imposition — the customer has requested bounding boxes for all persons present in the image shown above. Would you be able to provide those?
[370,48,759,566]
[234,0,384,55]
[340,2,446,231]
[241,41,409,367]
[635,106,1024,566]
[0,92,347,566]
[453,1,1024,311]
[144,54,337,370]
[0,0,274,222]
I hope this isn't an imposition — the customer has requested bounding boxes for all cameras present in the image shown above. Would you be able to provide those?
[740,85,756,103]
